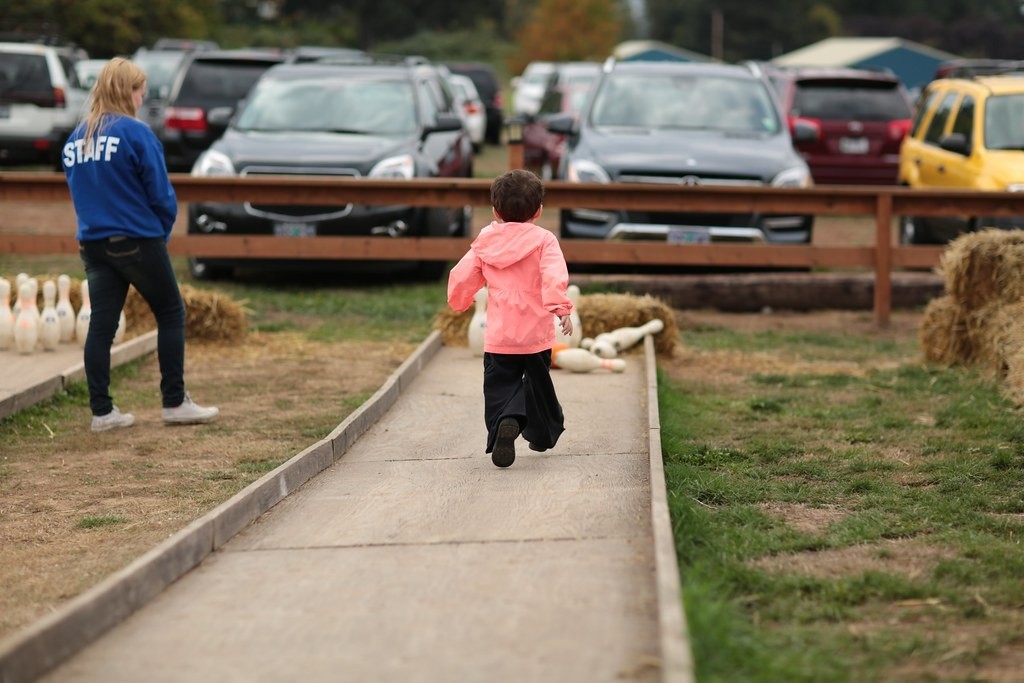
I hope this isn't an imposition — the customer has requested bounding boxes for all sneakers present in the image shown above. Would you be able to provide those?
[91,404,134,431]
[163,391,220,422]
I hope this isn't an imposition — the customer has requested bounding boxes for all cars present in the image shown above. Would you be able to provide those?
[562,54,814,275]
[767,63,913,202]
[0,36,1022,167]
[186,51,477,281]
[893,71,1023,245]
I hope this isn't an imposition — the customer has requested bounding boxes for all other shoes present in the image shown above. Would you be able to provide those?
[529,441,547,452]
[492,417,520,467]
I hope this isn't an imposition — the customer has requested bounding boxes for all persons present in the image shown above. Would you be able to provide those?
[448,170,573,467]
[62,57,219,432]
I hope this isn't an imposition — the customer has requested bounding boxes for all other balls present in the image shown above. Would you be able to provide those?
[551,343,570,369]
